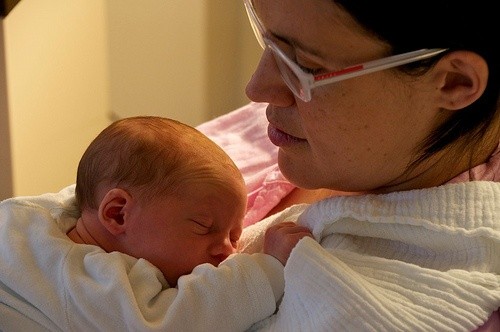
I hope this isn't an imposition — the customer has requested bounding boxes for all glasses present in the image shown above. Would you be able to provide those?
[243,0,447,103]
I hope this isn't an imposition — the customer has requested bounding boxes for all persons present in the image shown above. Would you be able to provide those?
[1,113,316,331]
[195,0,500,331]
